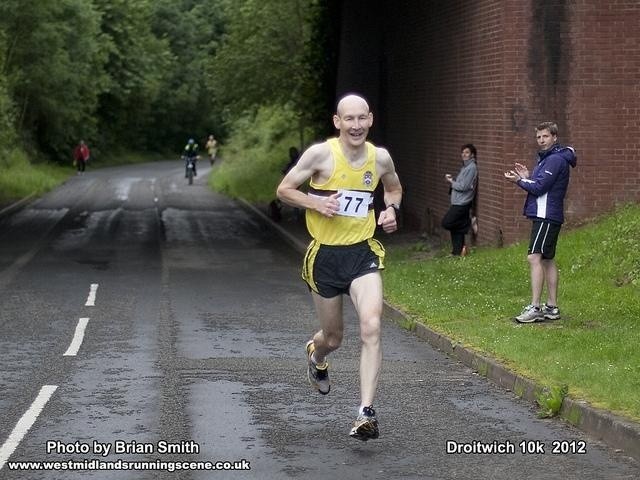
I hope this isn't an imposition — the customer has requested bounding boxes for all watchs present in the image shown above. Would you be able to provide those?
[386,203,400,214]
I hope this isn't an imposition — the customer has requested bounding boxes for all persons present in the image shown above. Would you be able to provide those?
[281,146,302,223]
[503,120,577,323]
[181,138,202,178]
[275,92,405,442]
[440,142,478,257]
[73,140,90,177]
[204,135,220,168]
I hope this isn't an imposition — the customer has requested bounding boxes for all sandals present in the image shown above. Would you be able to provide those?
[471,216,478,236]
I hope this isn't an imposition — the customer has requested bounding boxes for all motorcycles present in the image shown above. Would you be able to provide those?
[181,153,201,186]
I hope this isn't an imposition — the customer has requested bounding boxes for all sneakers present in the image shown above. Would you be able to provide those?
[348,411,379,442]
[542,303,561,320]
[305,339,331,396]
[514,304,545,323]
